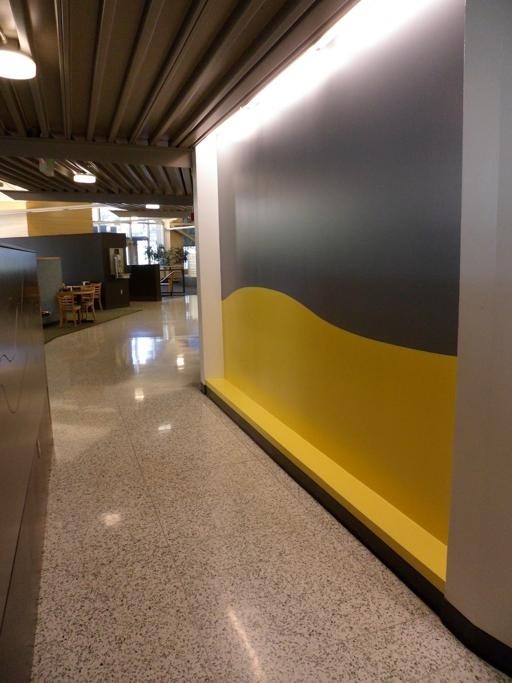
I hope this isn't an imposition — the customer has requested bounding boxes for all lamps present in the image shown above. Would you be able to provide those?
[0,33,42,83]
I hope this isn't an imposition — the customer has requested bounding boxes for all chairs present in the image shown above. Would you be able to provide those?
[53,279,105,327]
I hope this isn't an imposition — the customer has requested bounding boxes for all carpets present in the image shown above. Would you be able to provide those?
[42,304,145,344]
